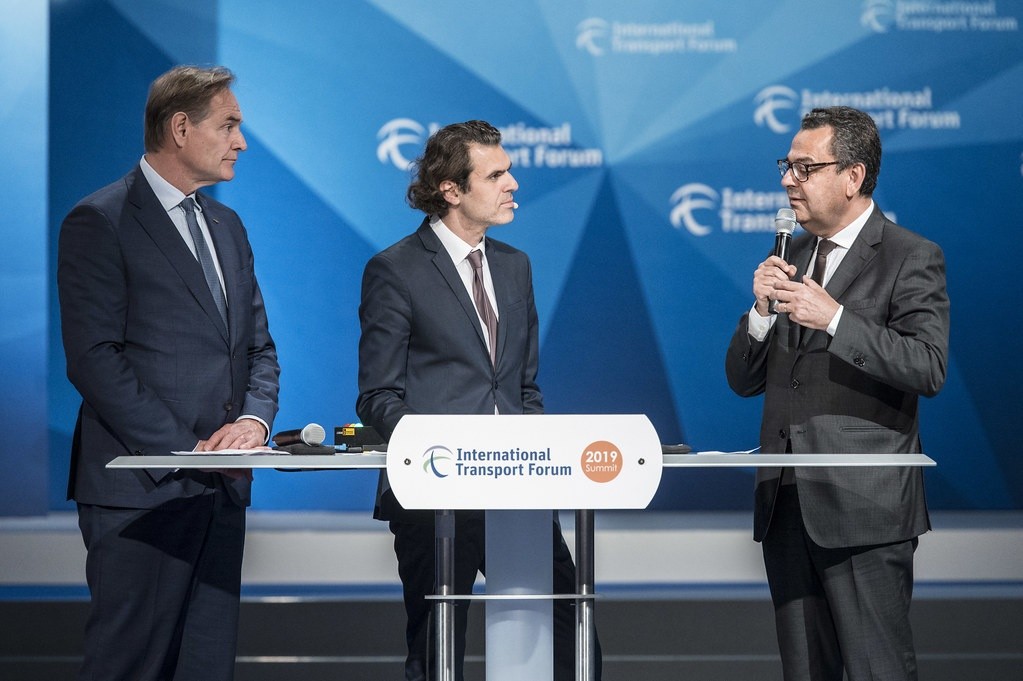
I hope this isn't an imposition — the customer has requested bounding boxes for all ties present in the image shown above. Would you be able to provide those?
[810,239,837,287]
[466,250,498,367]
[179,197,229,333]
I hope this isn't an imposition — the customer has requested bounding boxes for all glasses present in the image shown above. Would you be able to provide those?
[777,157,843,183]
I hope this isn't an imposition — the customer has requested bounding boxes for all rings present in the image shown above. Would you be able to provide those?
[784,303,788,312]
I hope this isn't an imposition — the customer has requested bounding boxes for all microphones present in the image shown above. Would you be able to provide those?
[767,208,797,313]
[512,202,519,210]
[271,423,326,446]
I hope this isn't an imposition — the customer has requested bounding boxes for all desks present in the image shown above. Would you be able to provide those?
[106,453,937,681]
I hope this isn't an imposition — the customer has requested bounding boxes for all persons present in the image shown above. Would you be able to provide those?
[56,65,281,681]
[357,120,603,681]
[725,105,949,681]
